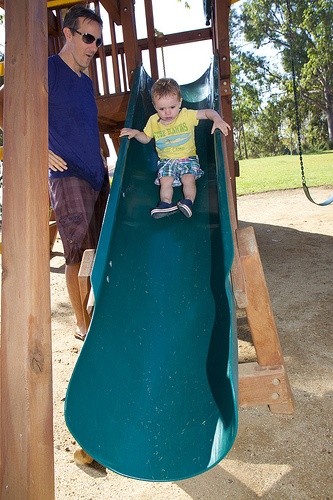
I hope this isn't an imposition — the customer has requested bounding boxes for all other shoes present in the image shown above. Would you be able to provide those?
[74,332,87,342]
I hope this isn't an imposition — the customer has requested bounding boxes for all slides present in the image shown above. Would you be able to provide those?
[64,50,239,482]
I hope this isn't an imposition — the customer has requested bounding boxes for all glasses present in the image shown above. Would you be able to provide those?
[68,27,102,48]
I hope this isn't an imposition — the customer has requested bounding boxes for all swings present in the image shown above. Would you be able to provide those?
[286,0,333,206]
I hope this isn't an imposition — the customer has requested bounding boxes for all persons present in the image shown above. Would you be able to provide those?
[119,78,231,219]
[0,6,111,340]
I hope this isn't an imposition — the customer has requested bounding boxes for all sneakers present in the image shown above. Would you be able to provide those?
[151,201,178,220]
[177,199,193,219]
